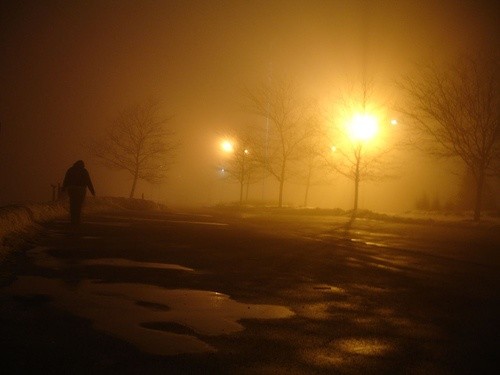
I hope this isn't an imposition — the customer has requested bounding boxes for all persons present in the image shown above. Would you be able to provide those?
[61,159,96,226]
